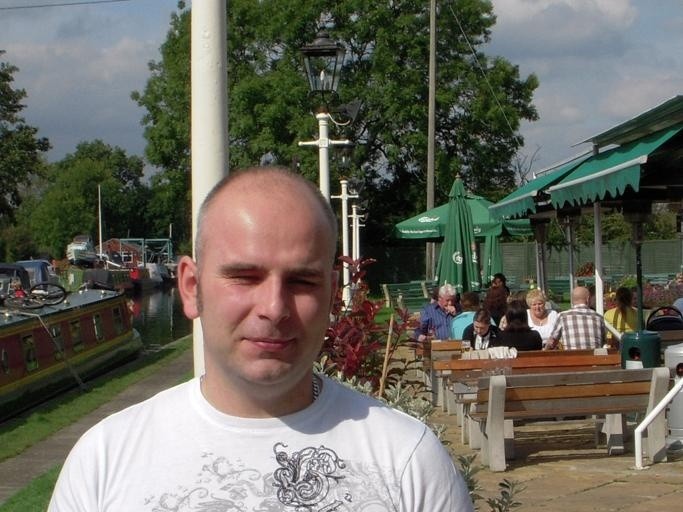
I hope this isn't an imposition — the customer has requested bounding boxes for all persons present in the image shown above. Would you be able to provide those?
[670,299,683,321]
[544,286,605,352]
[40,164,474,512]
[417,275,559,354]
[603,286,639,351]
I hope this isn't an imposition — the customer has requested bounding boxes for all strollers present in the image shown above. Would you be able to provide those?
[645,305,682,361]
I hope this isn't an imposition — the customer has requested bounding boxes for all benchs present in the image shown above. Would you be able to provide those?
[382,273,676,307]
[415,330,683,473]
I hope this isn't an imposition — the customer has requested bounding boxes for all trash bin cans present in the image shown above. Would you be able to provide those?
[620,331,661,368]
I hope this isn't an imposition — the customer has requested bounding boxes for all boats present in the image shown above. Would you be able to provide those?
[60,208,187,298]
[0,271,150,409]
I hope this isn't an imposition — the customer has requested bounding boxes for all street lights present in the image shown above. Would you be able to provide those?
[331,162,376,314]
[295,18,347,216]
